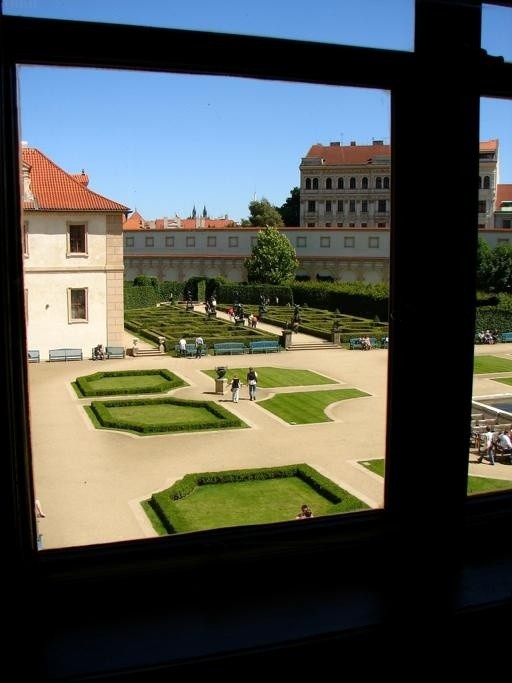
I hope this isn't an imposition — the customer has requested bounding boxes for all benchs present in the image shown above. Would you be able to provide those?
[213,343,244,356]
[380,337,389,349]
[48,348,83,362]
[470,413,512,465]
[349,338,378,350]
[475,334,498,344]
[175,344,208,357]
[501,333,512,343]
[27,350,40,363]
[249,341,280,354]
[92,347,125,359]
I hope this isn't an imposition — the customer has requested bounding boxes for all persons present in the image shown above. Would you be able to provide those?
[247,367,258,400]
[478,427,497,465]
[230,374,242,403]
[36,498,44,520]
[301,509,312,518]
[357,334,388,351]
[95,344,105,360]
[498,430,512,463]
[477,327,500,344]
[275,296,279,305]
[179,337,186,358]
[229,305,257,329]
[195,336,203,359]
[296,505,308,519]
